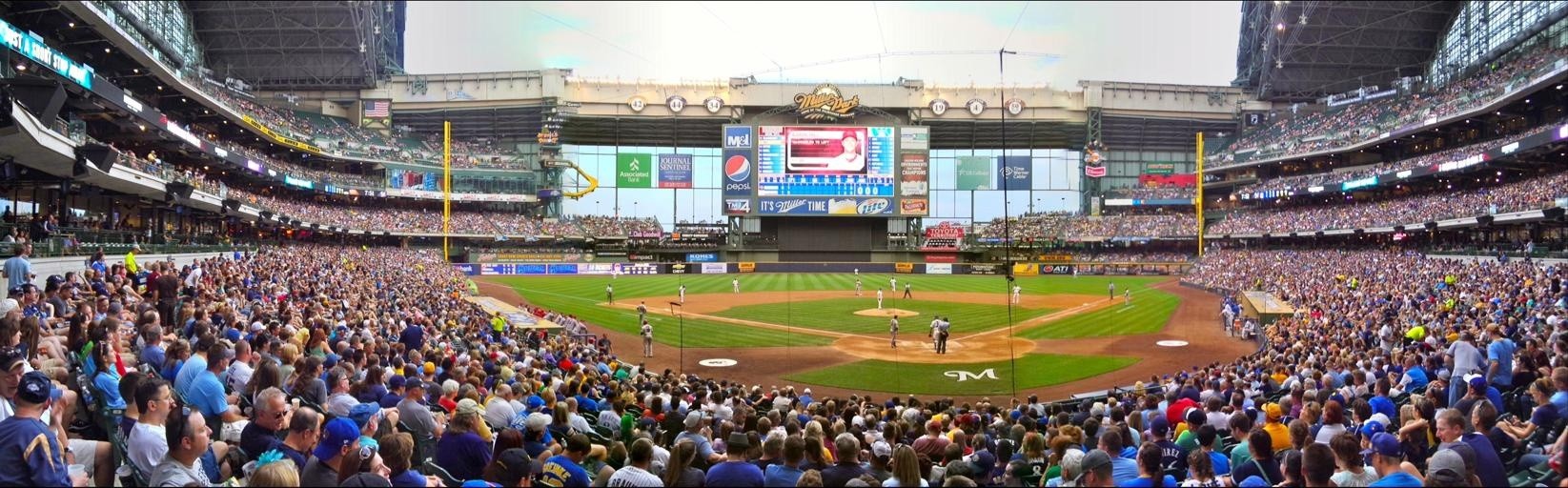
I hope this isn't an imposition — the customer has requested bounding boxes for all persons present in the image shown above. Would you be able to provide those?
[2,45,1567,484]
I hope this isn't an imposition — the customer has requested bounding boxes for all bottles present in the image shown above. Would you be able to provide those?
[561,438,567,449]
[1547,430,1556,443]
[75,367,93,403]
[1525,441,1538,454]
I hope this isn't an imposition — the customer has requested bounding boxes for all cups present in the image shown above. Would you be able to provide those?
[292,398,300,409]
[67,463,85,478]
[116,465,133,477]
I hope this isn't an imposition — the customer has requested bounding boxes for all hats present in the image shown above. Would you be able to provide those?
[1359,412,1400,455]
[1245,402,1282,420]
[1428,441,1476,482]
[10,275,65,295]
[455,398,485,415]
[852,399,994,474]
[514,362,526,370]
[1463,373,1486,392]
[251,322,267,331]
[685,410,706,427]
[491,353,499,358]
[388,362,435,389]
[323,326,346,366]
[526,396,552,431]
[1486,324,1498,333]
[804,388,811,393]
[0,346,51,402]
[1075,449,1111,481]
[729,432,750,447]
[496,448,542,478]
[313,402,379,460]
[1151,408,1207,432]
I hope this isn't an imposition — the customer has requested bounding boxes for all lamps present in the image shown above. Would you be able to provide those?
[359,38,367,53]
[1277,16,1284,32]
[385,1,394,13]
[374,19,382,34]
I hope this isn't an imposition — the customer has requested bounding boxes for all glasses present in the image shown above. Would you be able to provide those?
[276,411,287,419]
[99,340,106,356]
[180,406,192,431]
